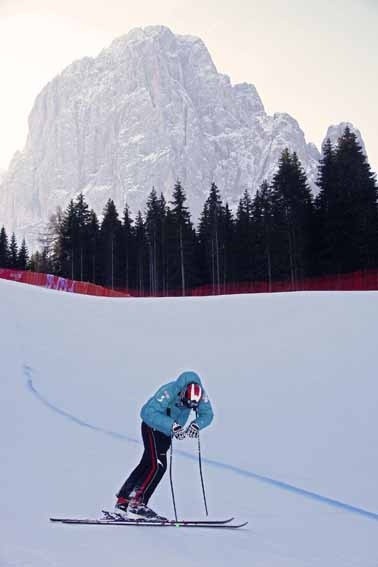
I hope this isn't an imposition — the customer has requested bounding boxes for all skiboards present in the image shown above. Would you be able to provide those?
[49,512,248,528]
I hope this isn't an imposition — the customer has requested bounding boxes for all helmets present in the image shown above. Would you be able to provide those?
[186,383,202,403]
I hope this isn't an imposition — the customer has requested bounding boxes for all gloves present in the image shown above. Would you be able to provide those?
[185,421,200,438]
[173,423,185,440]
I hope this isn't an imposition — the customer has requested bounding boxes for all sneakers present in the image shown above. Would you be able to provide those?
[127,497,157,518]
[115,497,129,513]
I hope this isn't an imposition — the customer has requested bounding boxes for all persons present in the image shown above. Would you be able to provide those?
[115,371,214,521]
